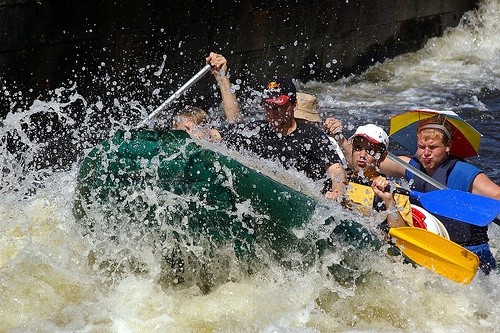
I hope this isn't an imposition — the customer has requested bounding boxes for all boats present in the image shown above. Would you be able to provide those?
[73,130,418,288]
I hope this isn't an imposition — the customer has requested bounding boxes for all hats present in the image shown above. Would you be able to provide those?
[261,77,295,106]
[293,93,323,123]
[348,123,389,152]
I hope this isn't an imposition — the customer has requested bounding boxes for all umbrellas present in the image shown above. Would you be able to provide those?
[389,106,483,160]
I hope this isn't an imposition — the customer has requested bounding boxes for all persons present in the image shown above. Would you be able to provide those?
[378,117,499,277]
[294,91,355,172]
[172,50,246,136]
[322,123,414,234]
[193,77,347,203]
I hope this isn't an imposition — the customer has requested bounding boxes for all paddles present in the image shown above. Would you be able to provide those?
[386,150,500,227]
[131,60,213,131]
[397,186,500,228]
[342,204,480,287]
